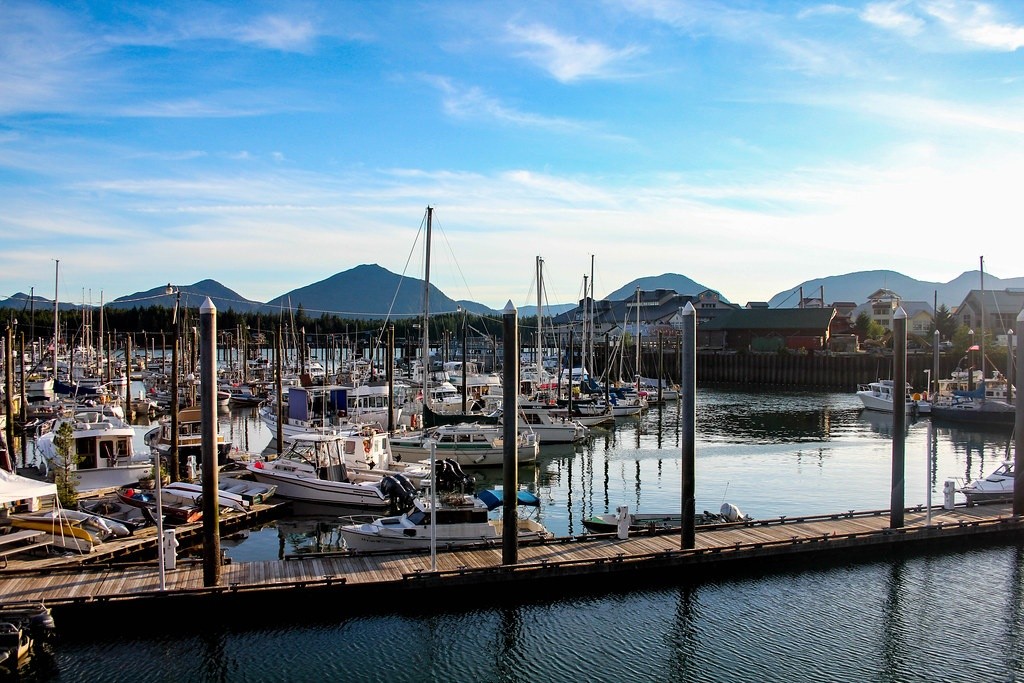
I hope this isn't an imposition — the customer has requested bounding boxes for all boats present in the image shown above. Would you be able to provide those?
[854,254,1024,424]
[961,461,1016,505]
[580,502,751,539]
[0,203,682,559]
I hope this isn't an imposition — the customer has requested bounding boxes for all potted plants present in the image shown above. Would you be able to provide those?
[51,422,86,511]
[140,477,156,490]
[152,464,168,487]
